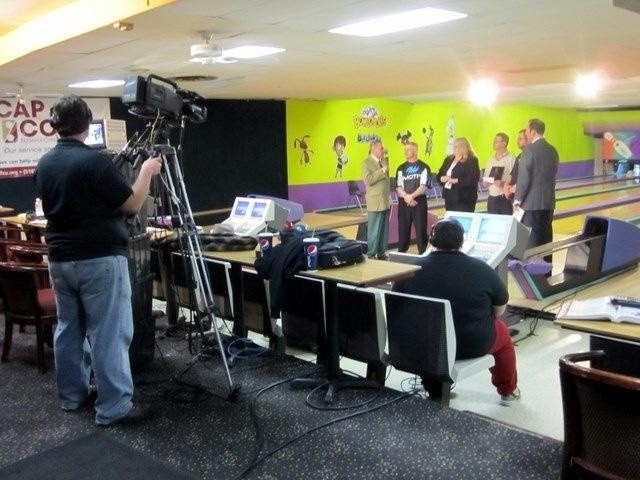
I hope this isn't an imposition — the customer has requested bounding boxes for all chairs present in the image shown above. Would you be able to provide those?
[385,291,495,407]
[560,351,640,479]
[0,220,59,374]
[335,282,388,385]
[426,173,443,201]
[280,272,330,365]
[172,250,204,329]
[228,266,285,352]
[346,181,367,212]
[200,257,237,334]
[389,178,399,223]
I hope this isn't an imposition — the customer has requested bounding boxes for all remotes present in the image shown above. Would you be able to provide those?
[611,296,640,307]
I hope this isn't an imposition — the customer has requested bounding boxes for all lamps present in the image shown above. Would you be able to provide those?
[190,31,222,58]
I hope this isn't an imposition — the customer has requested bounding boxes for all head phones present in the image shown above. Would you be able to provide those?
[426,218,466,245]
[49,98,92,130]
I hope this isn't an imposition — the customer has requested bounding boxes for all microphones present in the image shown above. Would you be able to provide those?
[385,158,389,179]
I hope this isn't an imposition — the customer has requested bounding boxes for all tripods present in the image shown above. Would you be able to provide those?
[111,144,243,400]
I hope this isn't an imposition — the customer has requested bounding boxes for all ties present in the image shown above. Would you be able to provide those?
[379,161,383,168]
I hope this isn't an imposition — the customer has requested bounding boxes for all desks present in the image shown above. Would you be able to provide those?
[144,224,423,287]
[554,271,640,378]
[303,213,361,228]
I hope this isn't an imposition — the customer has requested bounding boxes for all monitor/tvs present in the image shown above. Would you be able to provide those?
[443,211,479,240]
[230,197,253,219]
[250,199,289,231]
[83,120,108,150]
[475,212,534,261]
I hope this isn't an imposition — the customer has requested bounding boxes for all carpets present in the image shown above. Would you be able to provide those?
[0,432,201,480]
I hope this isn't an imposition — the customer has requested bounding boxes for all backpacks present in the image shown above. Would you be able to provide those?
[254,235,365,283]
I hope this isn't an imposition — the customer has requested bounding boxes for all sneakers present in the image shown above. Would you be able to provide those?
[501,386,521,406]
[377,252,388,260]
[112,398,157,423]
[63,384,98,412]
[368,255,377,259]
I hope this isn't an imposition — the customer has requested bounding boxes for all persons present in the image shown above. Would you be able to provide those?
[503,128,529,201]
[479,132,515,214]
[394,142,431,255]
[360,140,390,261]
[35,95,160,427]
[436,137,479,212]
[391,218,523,406]
[511,119,559,278]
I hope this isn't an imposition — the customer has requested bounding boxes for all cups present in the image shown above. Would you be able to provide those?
[257,233,272,264]
[304,237,320,273]
